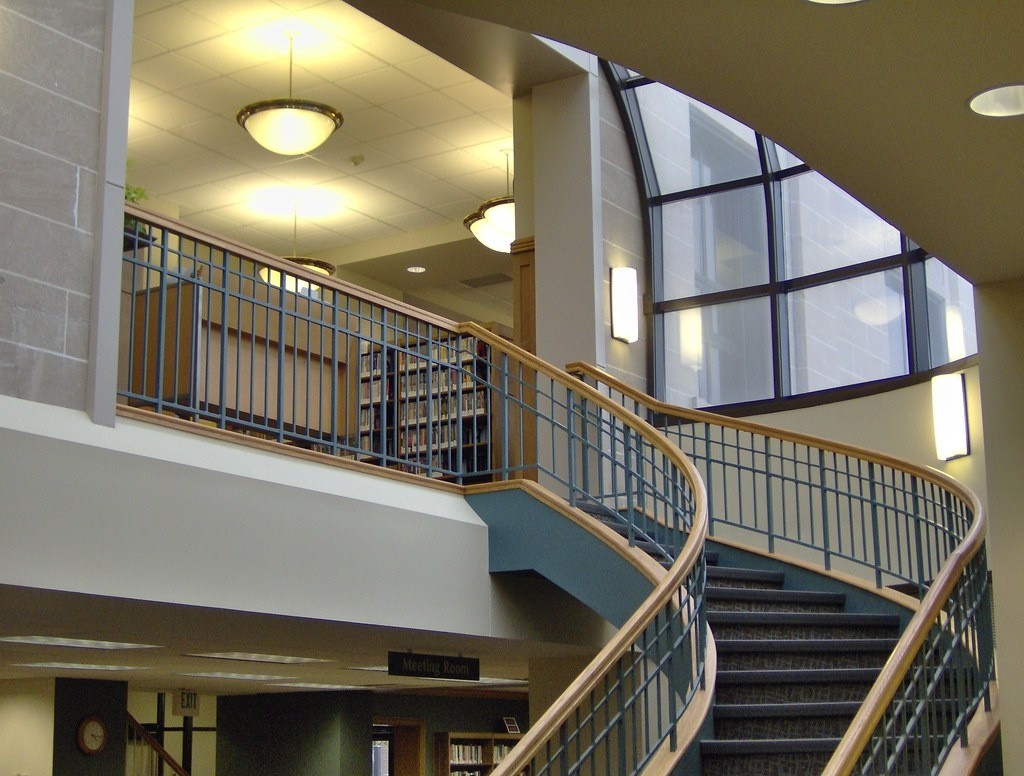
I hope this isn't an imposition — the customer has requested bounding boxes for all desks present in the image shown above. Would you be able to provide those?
[128,265,360,449]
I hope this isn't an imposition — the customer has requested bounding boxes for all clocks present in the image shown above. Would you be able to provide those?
[77,716,108,755]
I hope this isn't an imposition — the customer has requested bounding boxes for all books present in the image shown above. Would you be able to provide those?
[231,334,485,479]
[451,744,527,776]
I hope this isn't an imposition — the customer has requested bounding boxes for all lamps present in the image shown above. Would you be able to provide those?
[258,207,336,294]
[931,373,970,462]
[236,36,345,157]
[609,266,639,345]
[462,150,517,254]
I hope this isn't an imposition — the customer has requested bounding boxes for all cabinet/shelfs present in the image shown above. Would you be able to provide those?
[397,321,517,485]
[311,338,397,472]
[433,732,532,776]
